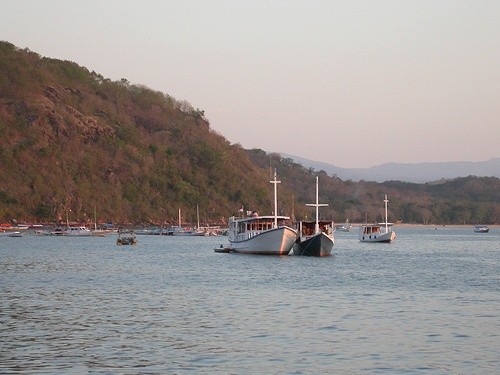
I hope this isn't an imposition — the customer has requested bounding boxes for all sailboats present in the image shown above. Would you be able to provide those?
[359,193,396,242]
[227,168,298,255]
[292,175,334,257]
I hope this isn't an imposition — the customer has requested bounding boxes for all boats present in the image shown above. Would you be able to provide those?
[7,232,23,237]
[473,226,491,232]
[117,231,137,246]
[213,246,230,253]
[336,219,352,232]
[34,205,227,238]
